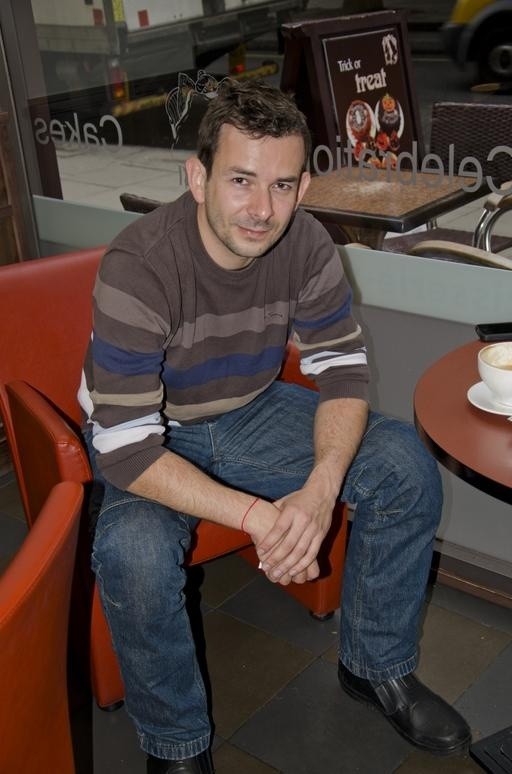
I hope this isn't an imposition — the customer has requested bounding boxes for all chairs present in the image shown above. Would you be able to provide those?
[406,181,512,270]
[0,244,352,710]
[0,481,84,774]
[369,100,512,262]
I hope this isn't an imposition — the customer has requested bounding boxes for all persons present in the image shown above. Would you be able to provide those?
[78,80,481,773]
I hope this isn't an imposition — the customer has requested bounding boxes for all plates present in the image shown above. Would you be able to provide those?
[345,100,377,147]
[466,380,512,416]
[375,96,405,139]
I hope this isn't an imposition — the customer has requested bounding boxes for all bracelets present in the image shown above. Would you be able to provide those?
[240,496,263,534]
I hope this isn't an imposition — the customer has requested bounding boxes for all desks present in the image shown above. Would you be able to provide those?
[293,164,500,249]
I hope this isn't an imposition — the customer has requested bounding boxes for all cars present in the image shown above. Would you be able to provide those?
[439,0,512,97]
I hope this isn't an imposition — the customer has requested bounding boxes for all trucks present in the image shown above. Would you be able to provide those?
[29,1,310,118]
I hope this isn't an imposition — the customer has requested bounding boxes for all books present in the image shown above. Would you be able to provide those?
[281,8,428,180]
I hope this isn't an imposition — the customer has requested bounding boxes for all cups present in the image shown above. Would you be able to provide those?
[476,341,512,398]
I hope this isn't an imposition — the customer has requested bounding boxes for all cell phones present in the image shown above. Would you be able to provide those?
[475,321,512,342]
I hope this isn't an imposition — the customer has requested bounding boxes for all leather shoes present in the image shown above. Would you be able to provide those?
[143,741,217,774]
[335,651,476,756]
